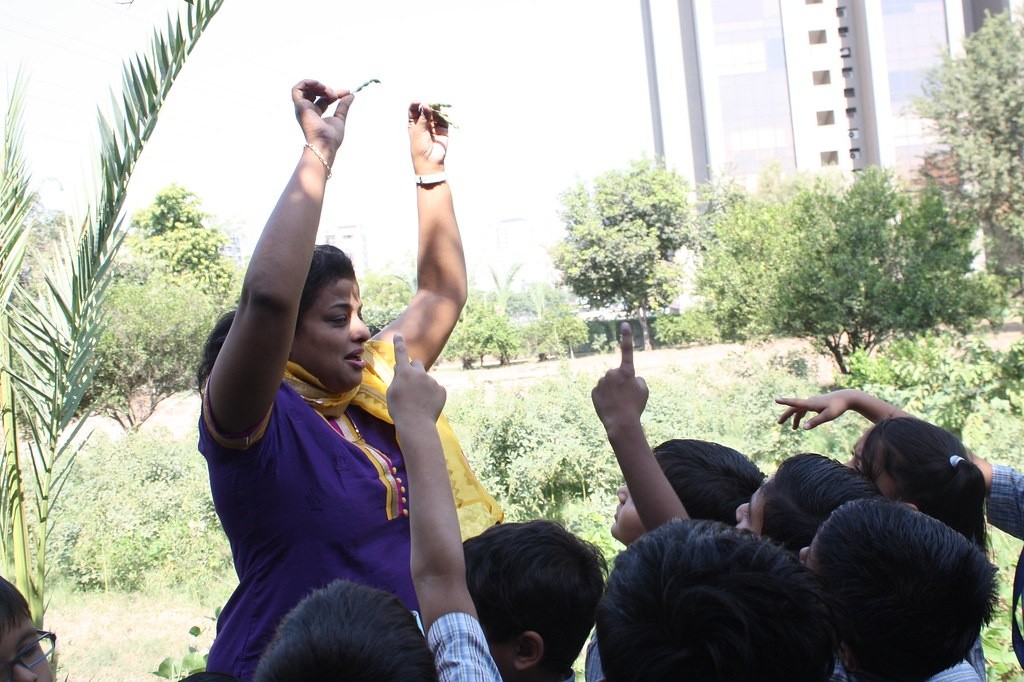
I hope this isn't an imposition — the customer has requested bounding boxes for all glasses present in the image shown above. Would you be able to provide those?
[0,629,56,682]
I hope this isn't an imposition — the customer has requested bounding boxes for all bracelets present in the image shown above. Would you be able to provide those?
[414,174,448,184]
[304,142,333,178]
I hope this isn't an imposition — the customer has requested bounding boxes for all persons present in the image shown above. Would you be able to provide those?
[0,573,60,682]
[253,321,1024,682]
[198,77,467,679]
